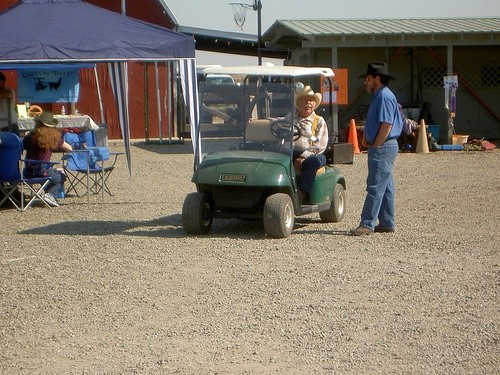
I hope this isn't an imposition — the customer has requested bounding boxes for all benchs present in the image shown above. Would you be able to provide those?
[244,120,326,177]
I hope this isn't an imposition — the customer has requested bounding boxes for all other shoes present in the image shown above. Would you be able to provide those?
[349,226,375,235]
[42,194,59,208]
[375,224,396,232]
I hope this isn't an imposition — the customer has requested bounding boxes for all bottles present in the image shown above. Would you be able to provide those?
[60,106,65,115]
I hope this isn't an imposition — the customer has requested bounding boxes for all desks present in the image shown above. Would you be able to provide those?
[19,115,99,138]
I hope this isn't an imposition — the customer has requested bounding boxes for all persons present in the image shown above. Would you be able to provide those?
[22,111,72,207]
[0,72,19,200]
[398,103,417,152]
[350,62,403,235]
[267,86,329,201]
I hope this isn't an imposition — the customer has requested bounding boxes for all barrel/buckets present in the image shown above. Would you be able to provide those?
[452,135,469,146]
[426,125,440,143]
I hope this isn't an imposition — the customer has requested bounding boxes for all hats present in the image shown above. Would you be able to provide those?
[34,110,59,127]
[295,84,322,111]
[357,61,398,84]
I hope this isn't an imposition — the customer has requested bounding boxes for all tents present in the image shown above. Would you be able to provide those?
[0,0,202,181]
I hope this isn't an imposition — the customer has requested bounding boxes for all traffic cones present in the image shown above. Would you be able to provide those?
[414,119,435,154]
[349,119,362,155]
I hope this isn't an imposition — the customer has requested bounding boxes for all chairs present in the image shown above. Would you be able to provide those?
[60,133,126,196]
[0,132,60,212]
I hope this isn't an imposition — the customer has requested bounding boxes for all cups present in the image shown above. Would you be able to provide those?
[24,102,30,114]
